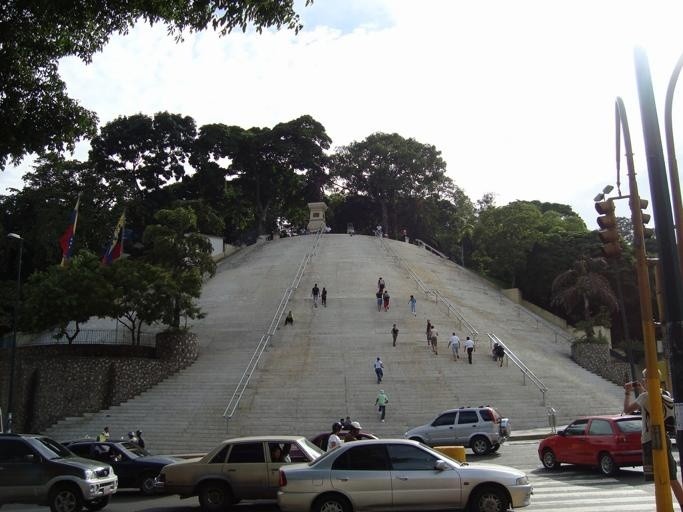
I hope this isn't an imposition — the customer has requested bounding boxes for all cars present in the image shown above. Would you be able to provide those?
[310,430,380,462]
[153,436,327,512]
[614,388,676,435]
[278,438,533,512]
[538,415,672,477]
[60,439,185,497]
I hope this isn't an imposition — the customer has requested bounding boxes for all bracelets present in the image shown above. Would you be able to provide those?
[624,390,632,396]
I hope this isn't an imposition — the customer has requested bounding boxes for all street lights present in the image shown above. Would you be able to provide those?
[593,184,640,398]
[6,233,24,433]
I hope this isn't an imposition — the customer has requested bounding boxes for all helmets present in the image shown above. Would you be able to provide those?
[333,422,345,431]
[351,421,362,430]
[127,431,135,436]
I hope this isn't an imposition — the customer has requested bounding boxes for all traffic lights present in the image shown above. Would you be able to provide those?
[629,196,654,241]
[595,199,621,261]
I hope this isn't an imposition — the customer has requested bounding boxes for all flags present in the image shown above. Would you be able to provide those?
[58,197,83,267]
[101,210,125,265]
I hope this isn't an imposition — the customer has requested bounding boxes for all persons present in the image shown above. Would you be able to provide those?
[375,276,390,312]
[492,343,498,360]
[407,295,417,319]
[326,413,360,452]
[271,447,280,462]
[425,320,473,364]
[623,365,682,509]
[319,287,327,307]
[110,449,121,461]
[99,424,145,447]
[284,309,294,325]
[309,283,319,308]
[374,389,388,422]
[544,403,558,432]
[496,345,504,367]
[280,442,292,462]
[373,356,384,384]
[390,323,399,347]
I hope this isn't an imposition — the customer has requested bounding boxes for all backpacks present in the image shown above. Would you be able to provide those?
[645,388,677,432]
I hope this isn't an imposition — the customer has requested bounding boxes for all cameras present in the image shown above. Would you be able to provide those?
[632,382,637,387]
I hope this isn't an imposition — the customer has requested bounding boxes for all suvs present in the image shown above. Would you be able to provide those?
[0,433,118,512]
[402,405,512,456]
